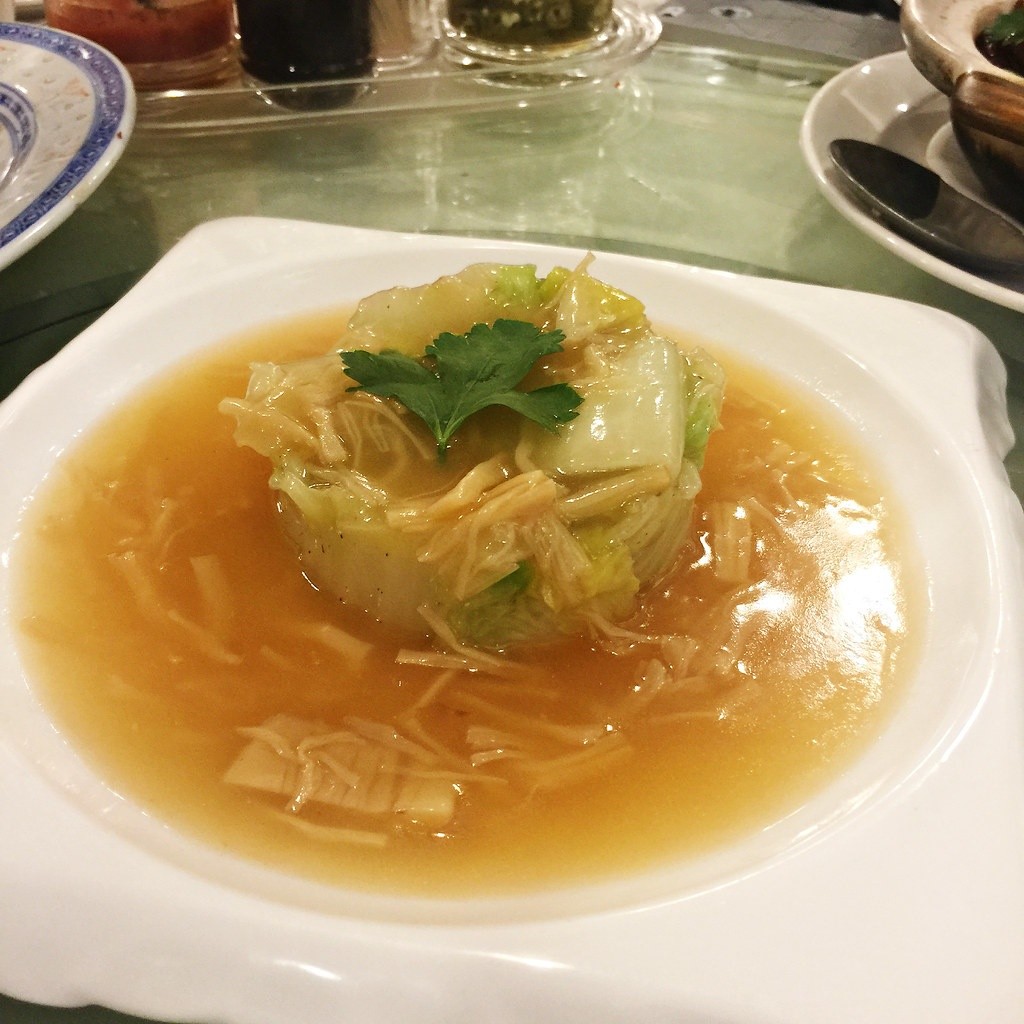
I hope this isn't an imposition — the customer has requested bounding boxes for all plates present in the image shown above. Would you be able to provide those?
[826,135,1024,271]
[798,49,1024,300]
[0,213,1024,1024]
[0,19,142,268]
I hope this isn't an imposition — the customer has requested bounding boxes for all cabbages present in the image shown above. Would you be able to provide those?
[213,267,725,655]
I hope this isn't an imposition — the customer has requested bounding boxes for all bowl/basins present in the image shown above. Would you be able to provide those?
[896,0,1023,170]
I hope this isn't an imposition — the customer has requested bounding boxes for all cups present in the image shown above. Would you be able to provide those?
[42,0,622,106]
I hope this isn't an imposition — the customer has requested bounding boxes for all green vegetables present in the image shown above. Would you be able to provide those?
[982,7,1024,45]
[340,318,584,464]
